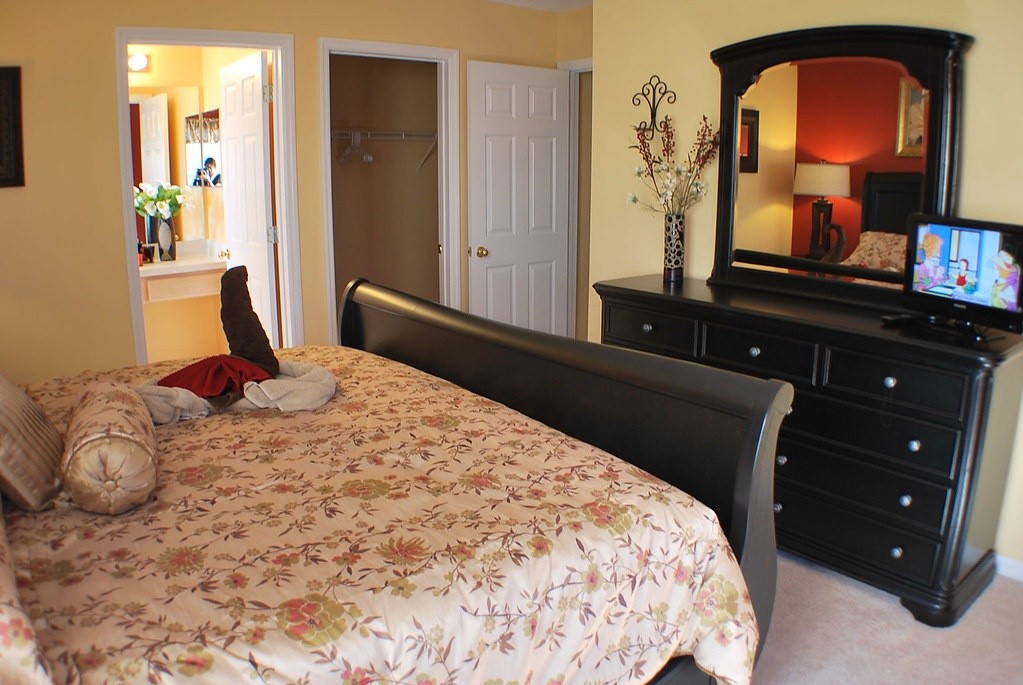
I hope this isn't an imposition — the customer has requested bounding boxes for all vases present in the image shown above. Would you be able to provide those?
[147,214,177,261]
[664,215,687,280]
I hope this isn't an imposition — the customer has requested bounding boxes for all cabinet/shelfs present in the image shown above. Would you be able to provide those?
[592,272,1023,628]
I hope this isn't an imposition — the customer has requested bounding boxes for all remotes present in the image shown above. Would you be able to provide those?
[881,315,913,325]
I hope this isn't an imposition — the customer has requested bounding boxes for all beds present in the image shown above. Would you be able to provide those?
[0,264,796,685]
[824,171,923,290]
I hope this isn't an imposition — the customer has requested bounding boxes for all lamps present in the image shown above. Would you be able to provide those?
[792,159,850,256]
[128,53,152,74]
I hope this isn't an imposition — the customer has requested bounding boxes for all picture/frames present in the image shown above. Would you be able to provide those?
[895,77,928,158]
[739,107,760,172]
[0,65,25,188]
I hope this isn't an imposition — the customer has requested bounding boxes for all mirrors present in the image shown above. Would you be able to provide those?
[184,108,221,187]
[710,24,974,321]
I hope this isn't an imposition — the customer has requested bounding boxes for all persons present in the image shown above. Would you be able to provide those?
[193,158,221,187]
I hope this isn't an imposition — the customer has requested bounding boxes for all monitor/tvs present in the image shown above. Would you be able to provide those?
[903,212,1023,342]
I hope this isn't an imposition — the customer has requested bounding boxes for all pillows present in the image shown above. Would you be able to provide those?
[0,514,51,685]
[60,385,160,518]
[0,373,69,512]
[839,232,908,272]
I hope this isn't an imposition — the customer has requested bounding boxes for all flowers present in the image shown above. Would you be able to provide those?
[627,116,721,217]
[133,177,198,221]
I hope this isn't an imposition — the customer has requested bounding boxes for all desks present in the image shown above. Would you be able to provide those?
[139,240,231,365]
